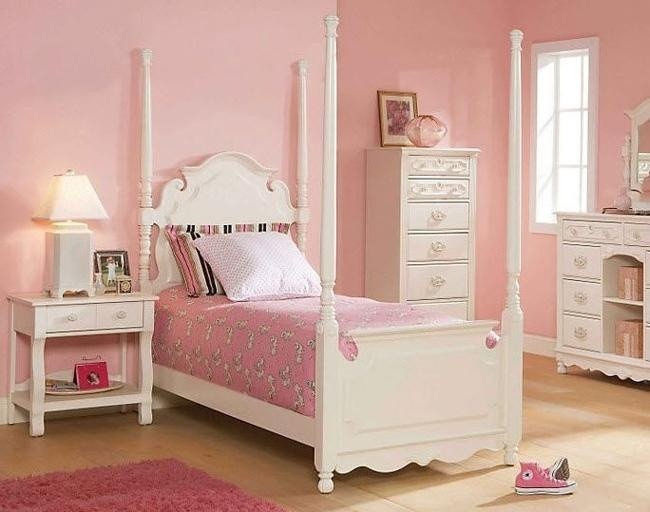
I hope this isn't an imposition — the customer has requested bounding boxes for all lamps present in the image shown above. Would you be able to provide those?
[31,169,109,299]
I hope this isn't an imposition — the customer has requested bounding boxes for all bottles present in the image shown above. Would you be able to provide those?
[93,272,107,294]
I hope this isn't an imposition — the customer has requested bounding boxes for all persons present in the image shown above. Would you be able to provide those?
[104,257,119,287]
[89,371,100,385]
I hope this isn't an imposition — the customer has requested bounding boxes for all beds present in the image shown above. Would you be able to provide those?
[138,151,523,495]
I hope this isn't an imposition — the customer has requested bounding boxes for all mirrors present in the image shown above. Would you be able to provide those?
[622,96,650,215]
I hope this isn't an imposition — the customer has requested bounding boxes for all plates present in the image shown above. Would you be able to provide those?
[46,380,124,396]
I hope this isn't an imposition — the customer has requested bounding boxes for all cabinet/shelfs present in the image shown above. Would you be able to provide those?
[365,147,481,324]
[553,211,650,383]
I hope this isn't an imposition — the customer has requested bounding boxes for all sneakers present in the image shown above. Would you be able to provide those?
[543,457,572,482]
[513,460,578,496]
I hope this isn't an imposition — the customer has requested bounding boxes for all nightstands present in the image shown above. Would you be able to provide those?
[5,294,161,438]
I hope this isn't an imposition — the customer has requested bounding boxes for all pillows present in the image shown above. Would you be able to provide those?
[163,223,323,302]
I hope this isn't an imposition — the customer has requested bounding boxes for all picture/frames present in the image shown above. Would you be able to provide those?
[75,361,109,390]
[93,250,132,294]
[377,91,418,147]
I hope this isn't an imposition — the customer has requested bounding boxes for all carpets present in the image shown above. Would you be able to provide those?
[0,457,290,512]
